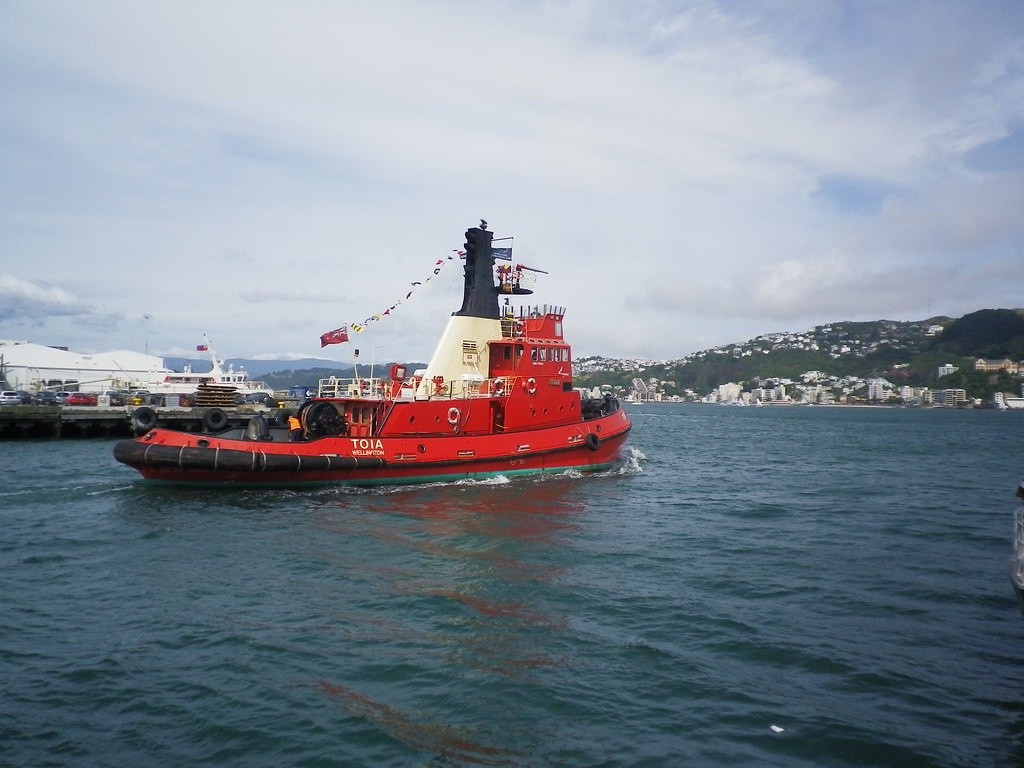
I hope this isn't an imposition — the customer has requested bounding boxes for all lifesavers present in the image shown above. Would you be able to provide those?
[514,320,525,335]
[447,407,461,424]
[361,379,373,394]
[276,410,296,428]
[585,432,601,450]
[130,406,157,433]
[526,377,537,394]
[494,379,504,394]
[204,409,227,431]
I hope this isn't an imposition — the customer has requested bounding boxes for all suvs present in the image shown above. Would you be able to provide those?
[0,390,20,405]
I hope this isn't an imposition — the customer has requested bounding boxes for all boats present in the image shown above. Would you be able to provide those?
[113,226,632,489]
[147,334,276,398]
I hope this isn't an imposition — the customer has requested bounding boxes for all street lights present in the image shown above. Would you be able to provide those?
[370,345,385,397]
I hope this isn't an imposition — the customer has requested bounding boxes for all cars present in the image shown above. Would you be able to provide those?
[65,391,88,405]
[103,390,143,406]
[129,390,150,398]
[18,391,31,404]
[34,390,56,405]
[54,391,71,404]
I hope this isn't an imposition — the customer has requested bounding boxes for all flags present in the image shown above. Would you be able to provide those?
[351,249,467,333]
[197,345,207,351]
[320,326,349,348]
